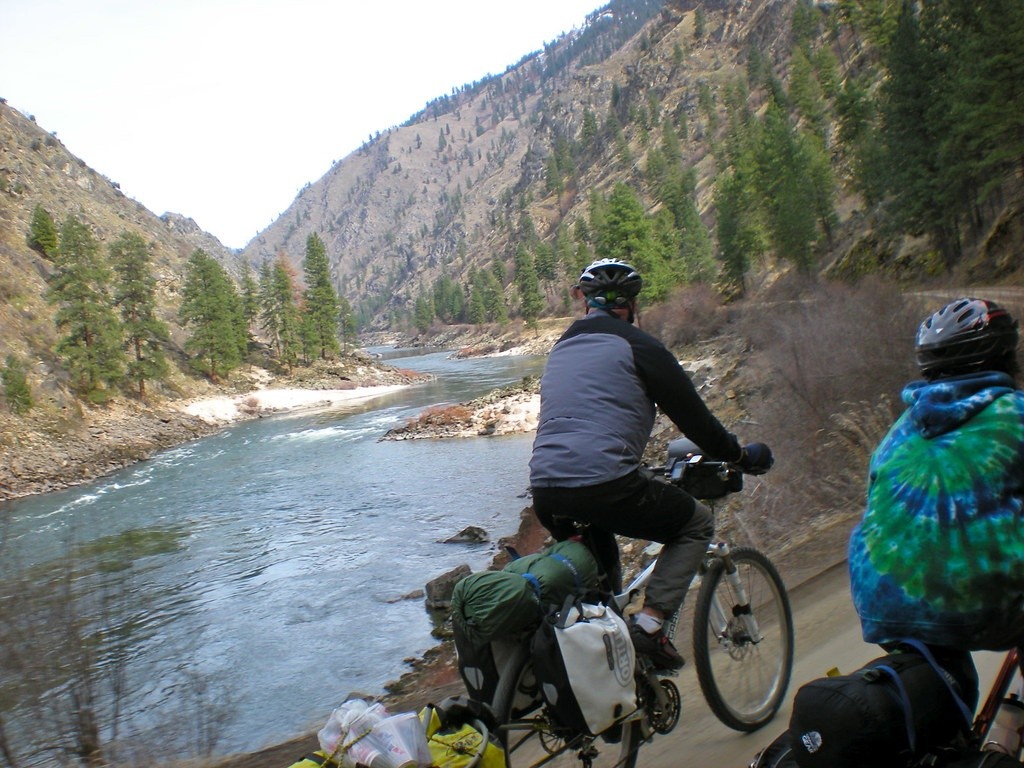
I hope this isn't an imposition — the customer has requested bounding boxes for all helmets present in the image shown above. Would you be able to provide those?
[579,259,642,305]
[913,298,1020,377]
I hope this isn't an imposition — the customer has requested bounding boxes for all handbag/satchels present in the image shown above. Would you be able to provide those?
[789,642,980,768]
[527,591,645,748]
[448,616,498,704]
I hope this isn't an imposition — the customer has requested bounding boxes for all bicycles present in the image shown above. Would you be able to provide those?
[879,638,1024,768]
[487,464,794,768]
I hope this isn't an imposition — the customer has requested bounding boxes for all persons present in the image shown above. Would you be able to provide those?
[527,258,773,669]
[847,298,1024,718]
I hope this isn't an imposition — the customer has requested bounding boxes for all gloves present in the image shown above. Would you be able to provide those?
[739,441,773,475]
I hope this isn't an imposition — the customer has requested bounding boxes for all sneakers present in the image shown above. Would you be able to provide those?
[629,622,685,670]
